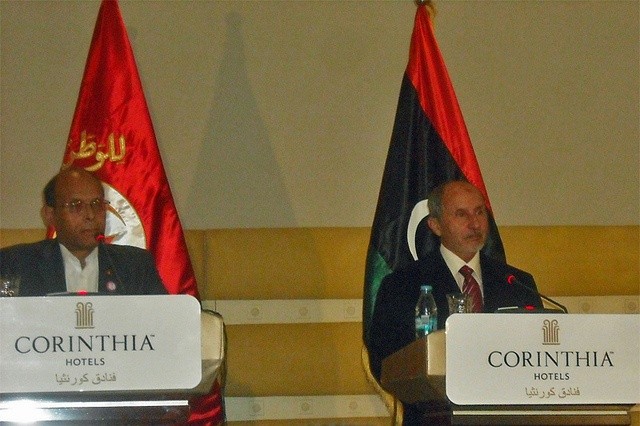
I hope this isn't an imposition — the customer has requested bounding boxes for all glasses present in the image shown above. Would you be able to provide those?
[52,199,110,210]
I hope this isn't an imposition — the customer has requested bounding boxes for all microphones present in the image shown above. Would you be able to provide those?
[94,231,127,295]
[503,271,568,313]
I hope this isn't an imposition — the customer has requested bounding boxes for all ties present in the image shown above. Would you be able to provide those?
[459,266,482,313]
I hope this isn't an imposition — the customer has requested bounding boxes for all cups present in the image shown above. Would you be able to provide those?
[446,293,475,316]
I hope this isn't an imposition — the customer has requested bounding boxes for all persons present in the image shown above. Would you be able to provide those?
[1,168,168,297]
[367,180,543,426]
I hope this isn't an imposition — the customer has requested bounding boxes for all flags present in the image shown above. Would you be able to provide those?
[46,0,225,425]
[362,0,508,345]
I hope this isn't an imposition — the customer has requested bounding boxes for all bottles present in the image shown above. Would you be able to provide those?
[413,284,438,342]
